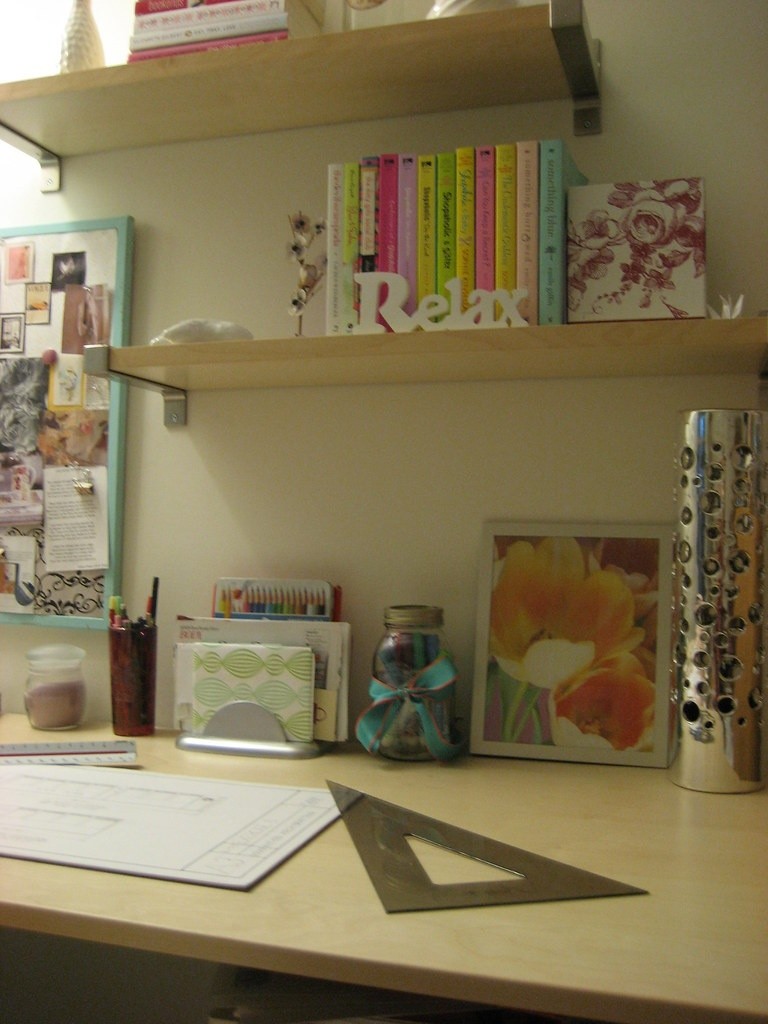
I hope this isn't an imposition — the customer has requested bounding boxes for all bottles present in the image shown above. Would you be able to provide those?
[372,605,455,762]
[60,0,105,72]
[24,646,86,730]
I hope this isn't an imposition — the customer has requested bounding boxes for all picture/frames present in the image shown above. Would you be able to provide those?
[0,215,135,632]
[466,521,677,771]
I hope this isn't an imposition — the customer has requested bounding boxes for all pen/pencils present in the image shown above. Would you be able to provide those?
[108,576,159,631]
[215,584,327,619]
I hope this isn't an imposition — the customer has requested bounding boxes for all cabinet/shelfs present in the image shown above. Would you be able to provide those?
[0,0,768,429]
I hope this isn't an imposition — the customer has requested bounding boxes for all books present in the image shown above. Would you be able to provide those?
[327,139,590,337]
[127,0,325,63]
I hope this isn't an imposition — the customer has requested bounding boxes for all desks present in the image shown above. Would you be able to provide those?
[0,711,768,1024]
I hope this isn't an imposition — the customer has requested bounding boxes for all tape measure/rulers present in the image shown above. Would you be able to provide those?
[0,737,139,768]
[322,776,652,917]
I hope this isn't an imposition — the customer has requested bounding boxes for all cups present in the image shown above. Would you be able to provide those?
[12,465,36,491]
[107,625,156,737]
